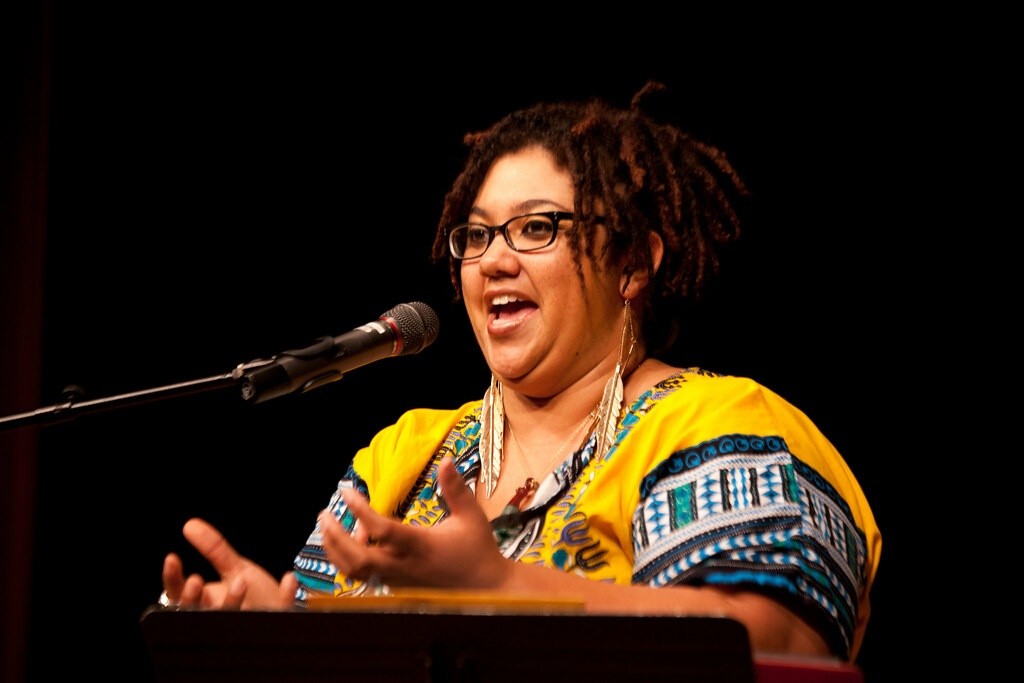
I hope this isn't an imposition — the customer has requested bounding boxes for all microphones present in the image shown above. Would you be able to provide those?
[241,300,440,405]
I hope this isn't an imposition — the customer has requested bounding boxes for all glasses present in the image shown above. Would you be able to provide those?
[443,211,606,260]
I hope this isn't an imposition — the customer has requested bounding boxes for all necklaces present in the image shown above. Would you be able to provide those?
[493,401,602,546]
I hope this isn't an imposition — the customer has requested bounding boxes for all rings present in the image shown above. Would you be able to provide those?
[159,590,170,607]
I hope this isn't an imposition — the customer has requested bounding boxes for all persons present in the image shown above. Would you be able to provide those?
[162,83,883,670]
